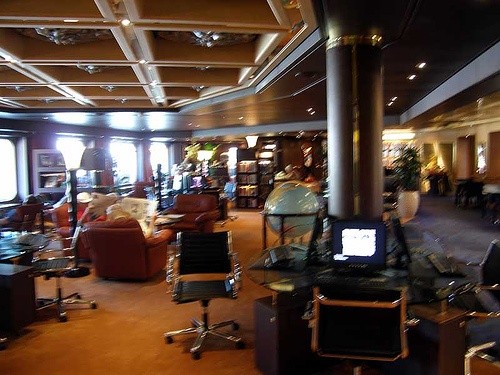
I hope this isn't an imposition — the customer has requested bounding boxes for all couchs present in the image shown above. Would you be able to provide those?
[54,201,106,262]
[84,217,172,281]
[163,193,221,245]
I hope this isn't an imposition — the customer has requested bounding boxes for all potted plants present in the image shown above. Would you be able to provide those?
[391,145,422,218]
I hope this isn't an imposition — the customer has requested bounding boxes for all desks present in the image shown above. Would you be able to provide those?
[247,242,479,375]
[0,229,51,335]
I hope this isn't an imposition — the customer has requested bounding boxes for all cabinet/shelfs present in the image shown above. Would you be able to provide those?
[235,148,275,210]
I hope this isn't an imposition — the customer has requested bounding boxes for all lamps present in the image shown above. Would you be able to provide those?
[59,147,113,278]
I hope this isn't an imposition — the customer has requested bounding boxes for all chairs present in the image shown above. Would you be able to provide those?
[28,222,96,322]
[9,202,43,229]
[164,231,244,359]
[457,237,500,318]
[303,285,419,375]
